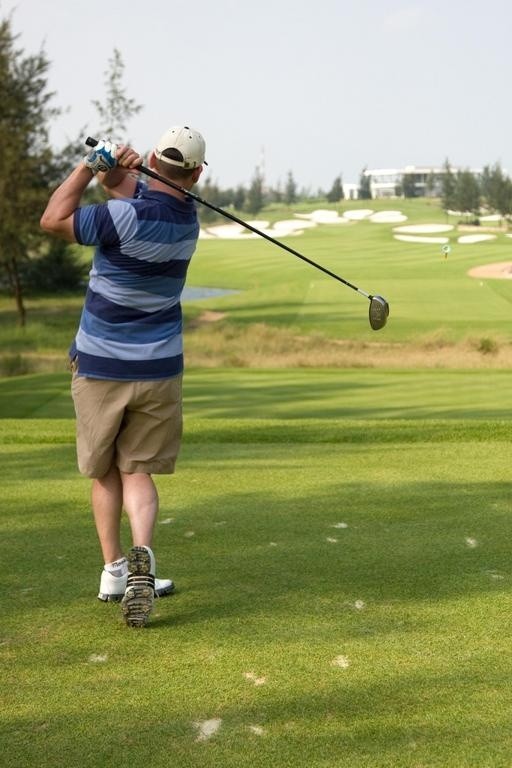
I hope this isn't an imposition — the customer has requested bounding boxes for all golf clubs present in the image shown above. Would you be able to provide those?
[84,136,390,330]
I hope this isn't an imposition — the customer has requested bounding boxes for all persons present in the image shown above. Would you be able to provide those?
[39,124,210,628]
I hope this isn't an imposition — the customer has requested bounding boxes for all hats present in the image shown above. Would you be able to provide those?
[153,125,208,171]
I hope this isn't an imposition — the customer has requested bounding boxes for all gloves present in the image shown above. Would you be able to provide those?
[83,139,118,176]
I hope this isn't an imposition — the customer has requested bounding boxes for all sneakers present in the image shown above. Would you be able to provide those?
[121,545,155,628]
[96,571,175,603]
